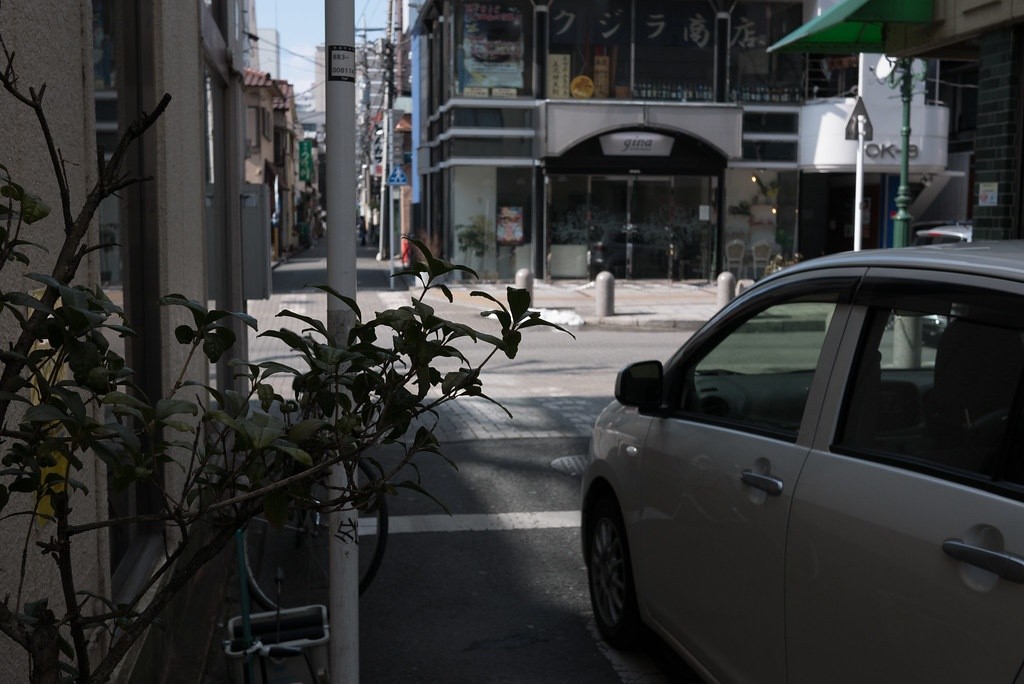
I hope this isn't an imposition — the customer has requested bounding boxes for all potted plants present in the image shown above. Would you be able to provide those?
[549,205,620,279]
[452,214,496,280]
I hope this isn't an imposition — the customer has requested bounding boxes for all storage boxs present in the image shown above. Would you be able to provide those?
[221,603,331,684]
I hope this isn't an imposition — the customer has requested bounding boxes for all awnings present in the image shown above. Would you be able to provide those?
[766,0,932,54]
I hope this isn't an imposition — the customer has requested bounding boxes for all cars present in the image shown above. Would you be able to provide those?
[581,239,1024,684]
[511,223,684,277]
[911,225,973,346]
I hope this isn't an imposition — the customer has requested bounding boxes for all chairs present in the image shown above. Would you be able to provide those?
[874,380,961,453]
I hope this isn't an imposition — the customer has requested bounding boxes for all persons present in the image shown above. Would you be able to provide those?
[359,216,368,246]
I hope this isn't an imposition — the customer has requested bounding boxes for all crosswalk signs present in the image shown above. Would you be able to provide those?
[386,165,409,184]
